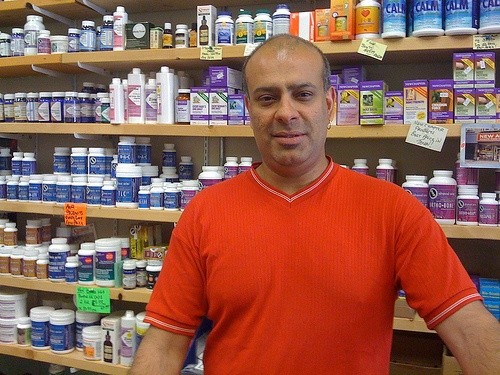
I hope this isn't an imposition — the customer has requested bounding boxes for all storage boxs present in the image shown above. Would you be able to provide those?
[359,81,386,125]
[208,87,236,125]
[341,64,365,84]
[384,91,404,125]
[474,51,496,88]
[315,9,330,41]
[495,88,500,124]
[125,21,156,50]
[290,11,315,41]
[475,88,496,124]
[244,105,251,125]
[188,85,209,125]
[429,79,454,124]
[403,79,429,124]
[329,74,342,126]
[453,89,476,123]
[338,84,359,126]
[202,68,211,86]
[228,94,245,125]
[386,85,390,91]
[208,66,243,90]
[331,0,360,40]
[453,52,474,89]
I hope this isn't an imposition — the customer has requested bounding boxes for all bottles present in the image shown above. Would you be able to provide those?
[355,0,500,38]
[9,287,151,367]
[0,214,164,292]
[0,5,128,58]
[351,152,500,224]
[0,66,191,133]
[162,20,197,50]
[215,4,292,45]
[0,136,252,213]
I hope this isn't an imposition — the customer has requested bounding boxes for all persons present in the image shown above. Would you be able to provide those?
[129,34,500,375]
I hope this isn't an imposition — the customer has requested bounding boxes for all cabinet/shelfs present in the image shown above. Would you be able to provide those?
[0,0,500,375]
